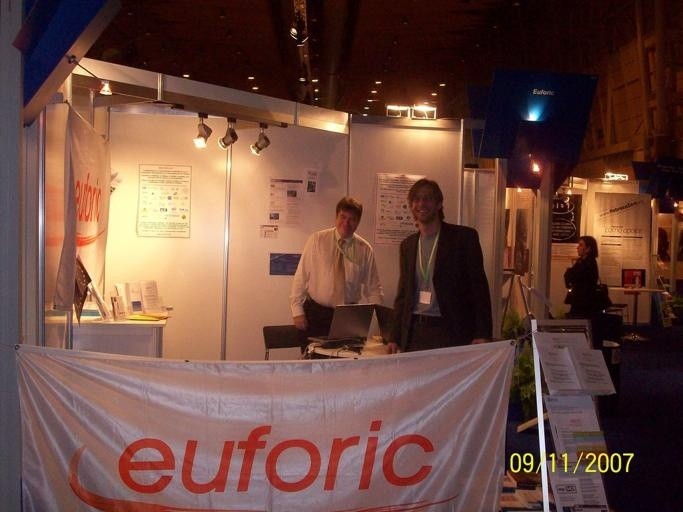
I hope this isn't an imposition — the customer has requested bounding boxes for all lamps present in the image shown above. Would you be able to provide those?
[66,13,311,155]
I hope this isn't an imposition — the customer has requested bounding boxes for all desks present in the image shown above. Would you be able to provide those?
[72,309,168,359]
[609,287,666,346]
[308,337,404,358]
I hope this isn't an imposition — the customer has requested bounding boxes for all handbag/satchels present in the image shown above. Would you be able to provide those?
[590,283,613,311]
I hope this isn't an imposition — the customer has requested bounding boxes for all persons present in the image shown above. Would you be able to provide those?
[287,196,387,360]
[384,176,494,353]
[562,234,604,349]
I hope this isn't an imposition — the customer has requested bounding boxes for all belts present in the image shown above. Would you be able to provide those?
[413,313,444,327]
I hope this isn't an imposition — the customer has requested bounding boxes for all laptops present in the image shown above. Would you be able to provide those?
[374,304,401,346]
[307,303,375,343]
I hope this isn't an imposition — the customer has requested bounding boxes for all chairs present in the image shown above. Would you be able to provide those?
[597,284,630,323]
[262,324,312,361]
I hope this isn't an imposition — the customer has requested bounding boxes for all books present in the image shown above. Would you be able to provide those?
[124,281,146,313]
[534,330,617,512]
[140,279,160,314]
[110,294,127,320]
[115,284,128,309]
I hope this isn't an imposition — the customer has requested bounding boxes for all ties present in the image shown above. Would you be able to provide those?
[331,239,347,308]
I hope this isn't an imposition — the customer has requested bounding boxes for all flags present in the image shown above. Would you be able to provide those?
[51,103,112,314]
[70,255,92,327]
